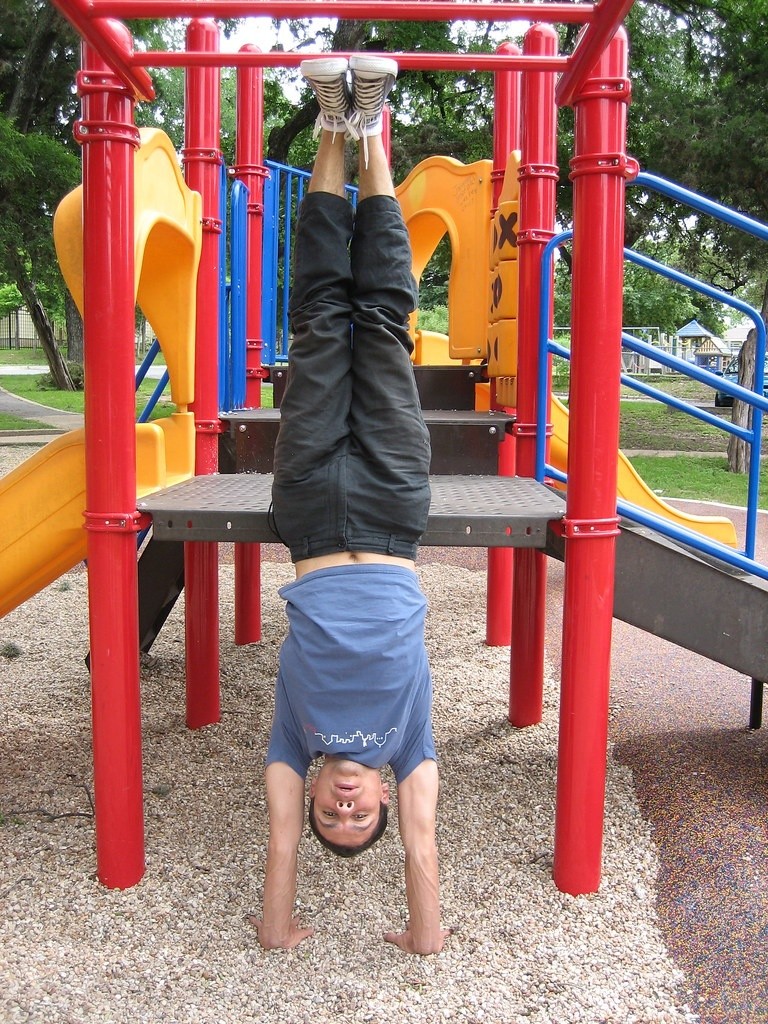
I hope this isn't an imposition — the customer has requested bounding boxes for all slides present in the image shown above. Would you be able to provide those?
[413,327,739,555]
[1,409,187,619]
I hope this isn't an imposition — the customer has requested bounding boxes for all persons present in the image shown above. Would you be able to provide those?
[246,56,451,955]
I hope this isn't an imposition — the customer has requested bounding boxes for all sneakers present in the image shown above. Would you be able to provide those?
[343,54,398,170]
[300,57,360,144]
